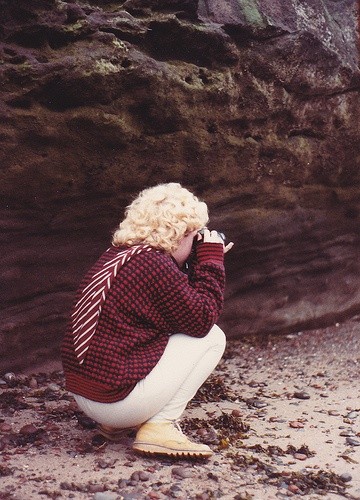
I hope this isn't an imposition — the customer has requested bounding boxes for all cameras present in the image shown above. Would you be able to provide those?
[192,230,227,260]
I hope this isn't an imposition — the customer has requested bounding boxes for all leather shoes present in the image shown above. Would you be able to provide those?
[133,424,212,458]
[98,426,140,437]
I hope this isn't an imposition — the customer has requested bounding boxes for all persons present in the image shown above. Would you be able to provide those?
[61,181,234,458]
